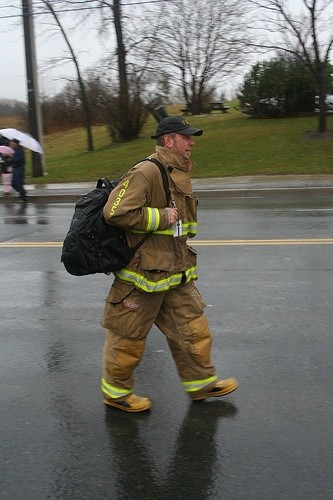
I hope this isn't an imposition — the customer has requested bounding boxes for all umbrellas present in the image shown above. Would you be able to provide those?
[0,146,14,155]
[0,128,44,154]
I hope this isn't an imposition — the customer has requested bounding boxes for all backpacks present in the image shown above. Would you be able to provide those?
[60,156,170,276]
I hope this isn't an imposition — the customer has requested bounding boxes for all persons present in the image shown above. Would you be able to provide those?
[3,138,26,196]
[100,116,239,412]
[0,155,13,195]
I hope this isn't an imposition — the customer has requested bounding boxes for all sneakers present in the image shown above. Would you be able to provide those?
[102,390,151,413]
[188,377,239,400]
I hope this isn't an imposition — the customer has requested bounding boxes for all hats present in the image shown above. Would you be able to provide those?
[150,115,203,139]
